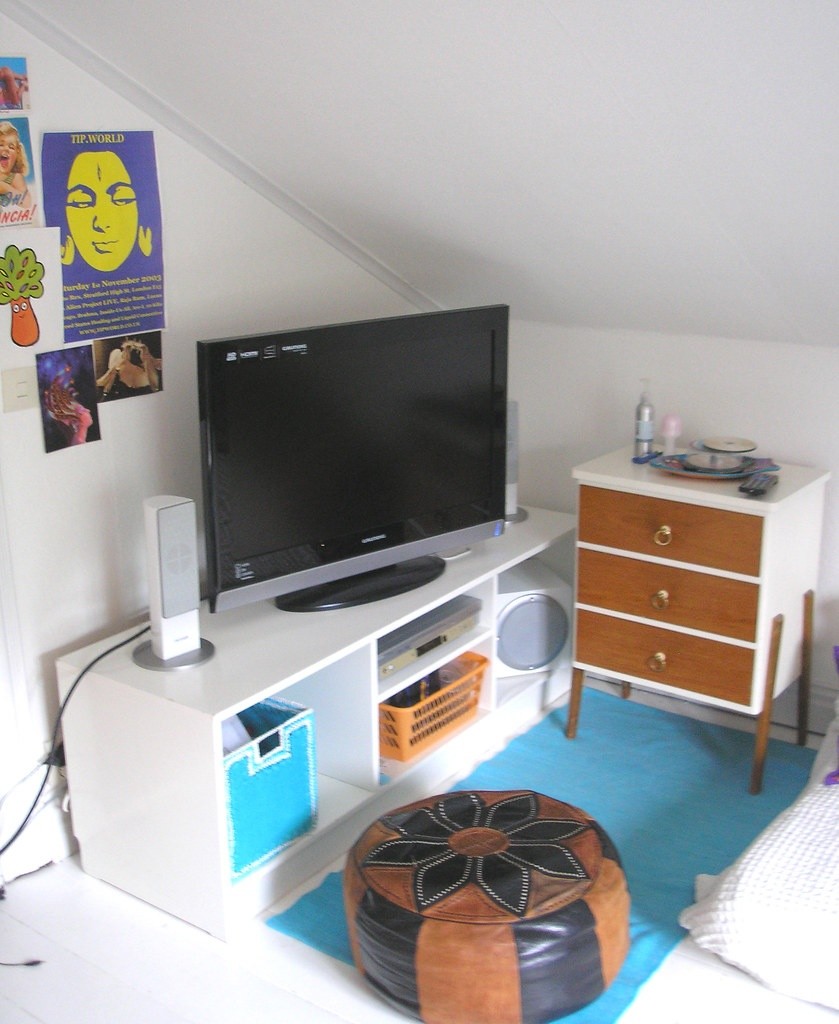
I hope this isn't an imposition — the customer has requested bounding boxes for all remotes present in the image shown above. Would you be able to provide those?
[739,473,778,496]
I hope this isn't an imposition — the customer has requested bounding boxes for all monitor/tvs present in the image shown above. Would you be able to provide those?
[195,303,510,613]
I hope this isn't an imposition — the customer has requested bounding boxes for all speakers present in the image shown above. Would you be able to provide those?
[143,495,201,661]
[495,560,572,678]
[506,401,516,521]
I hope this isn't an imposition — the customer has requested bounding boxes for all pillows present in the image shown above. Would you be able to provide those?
[678,697,839,1012]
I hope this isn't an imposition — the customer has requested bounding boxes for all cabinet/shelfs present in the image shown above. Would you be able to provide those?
[567,441,831,796]
[56,505,576,944]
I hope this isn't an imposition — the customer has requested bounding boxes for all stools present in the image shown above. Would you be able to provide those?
[344,789,629,1024]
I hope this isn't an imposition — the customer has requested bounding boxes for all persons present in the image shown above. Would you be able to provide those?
[0,67,29,105]
[0,120,30,211]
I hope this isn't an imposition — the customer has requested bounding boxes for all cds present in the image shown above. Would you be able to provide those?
[679,437,756,473]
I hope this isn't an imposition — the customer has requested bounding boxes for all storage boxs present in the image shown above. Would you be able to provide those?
[224,694,320,886]
[379,650,490,763]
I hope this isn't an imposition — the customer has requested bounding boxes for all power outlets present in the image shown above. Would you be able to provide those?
[44,734,66,786]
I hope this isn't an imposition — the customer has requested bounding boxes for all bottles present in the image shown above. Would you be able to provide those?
[634,393,655,457]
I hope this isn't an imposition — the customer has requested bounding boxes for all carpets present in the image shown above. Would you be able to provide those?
[266,685,817,1024]
